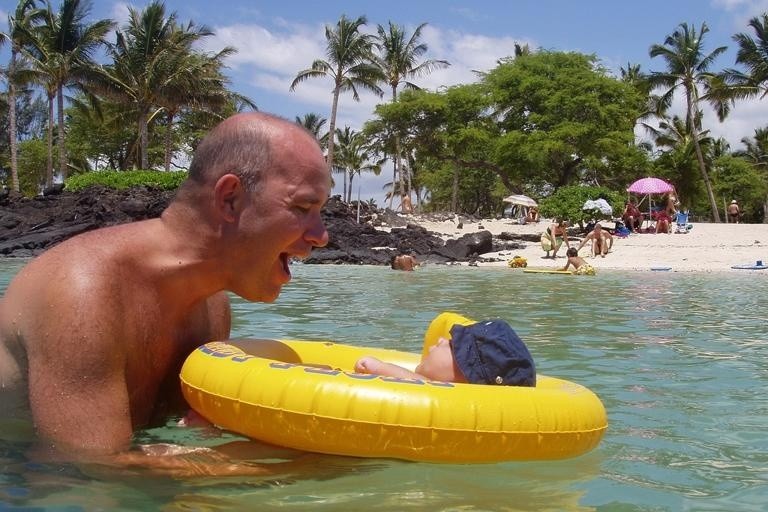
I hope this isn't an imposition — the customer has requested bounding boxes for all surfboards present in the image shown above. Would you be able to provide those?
[522,268,572,275]
[731,260,767,268]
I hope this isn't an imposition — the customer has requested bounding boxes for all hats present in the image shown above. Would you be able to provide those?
[449,317,536,388]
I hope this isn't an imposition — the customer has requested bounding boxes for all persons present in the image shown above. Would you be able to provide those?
[664,193,678,215]
[192,299,539,464]
[652,191,678,234]
[621,201,645,234]
[393,190,415,215]
[726,199,742,224]
[526,205,540,223]
[554,247,596,276]
[574,223,615,258]
[2,109,419,510]
[538,218,569,260]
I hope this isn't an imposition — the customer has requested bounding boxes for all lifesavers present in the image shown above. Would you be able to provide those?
[180,312,608,465]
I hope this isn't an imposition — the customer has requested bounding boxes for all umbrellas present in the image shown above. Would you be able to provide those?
[503,195,538,218]
[627,176,673,227]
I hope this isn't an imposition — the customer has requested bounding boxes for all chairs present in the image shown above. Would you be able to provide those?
[675,209,690,234]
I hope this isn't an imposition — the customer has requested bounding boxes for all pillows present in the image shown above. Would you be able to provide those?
[677,213,687,223]
